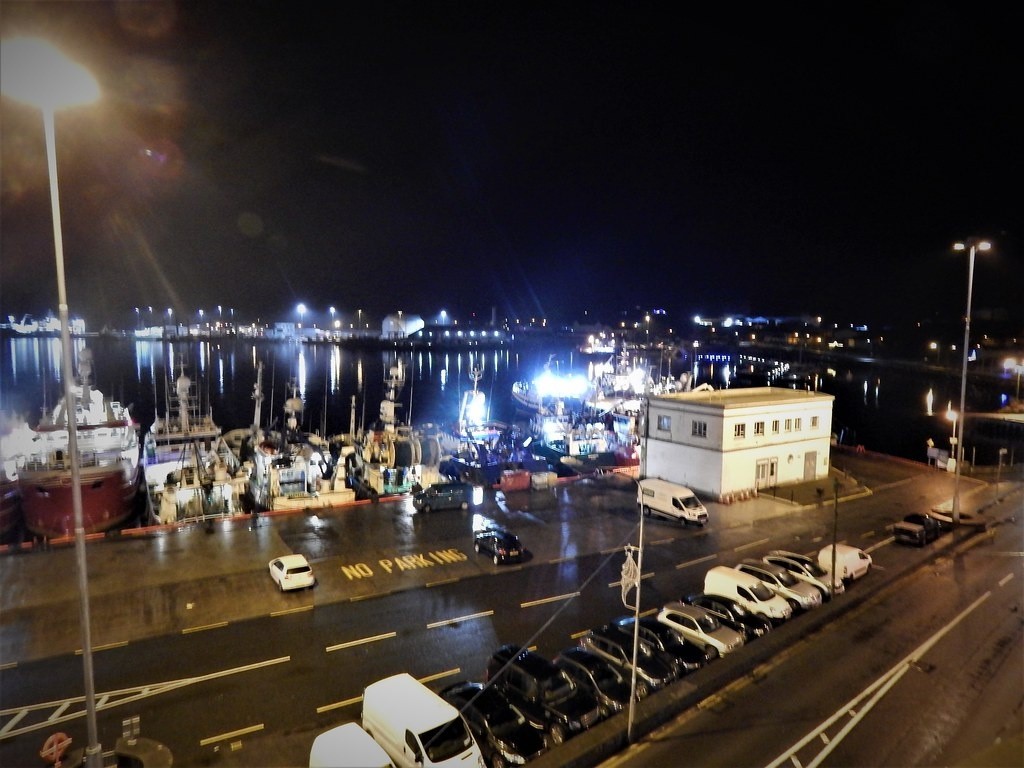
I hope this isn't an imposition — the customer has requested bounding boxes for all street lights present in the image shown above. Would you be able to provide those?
[0,39,115,767]
[952,237,991,538]
[561,455,639,748]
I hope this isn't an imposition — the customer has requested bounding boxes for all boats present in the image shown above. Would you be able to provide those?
[0,355,699,523]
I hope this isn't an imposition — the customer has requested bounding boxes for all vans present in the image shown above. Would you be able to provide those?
[413,482,474,513]
[817,544,874,587]
[308,722,394,768]
[361,673,488,768]
[704,566,793,627]
[636,478,710,529]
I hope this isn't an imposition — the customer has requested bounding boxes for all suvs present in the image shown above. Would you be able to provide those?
[577,623,686,692]
[766,549,845,598]
[735,559,823,615]
[490,643,609,746]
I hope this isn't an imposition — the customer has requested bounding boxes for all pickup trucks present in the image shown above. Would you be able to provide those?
[892,512,942,548]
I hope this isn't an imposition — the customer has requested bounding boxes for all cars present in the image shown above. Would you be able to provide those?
[658,601,745,665]
[475,527,527,567]
[441,681,550,768]
[550,648,640,713]
[269,553,317,595]
[619,616,712,674]
[682,592,769,644]
